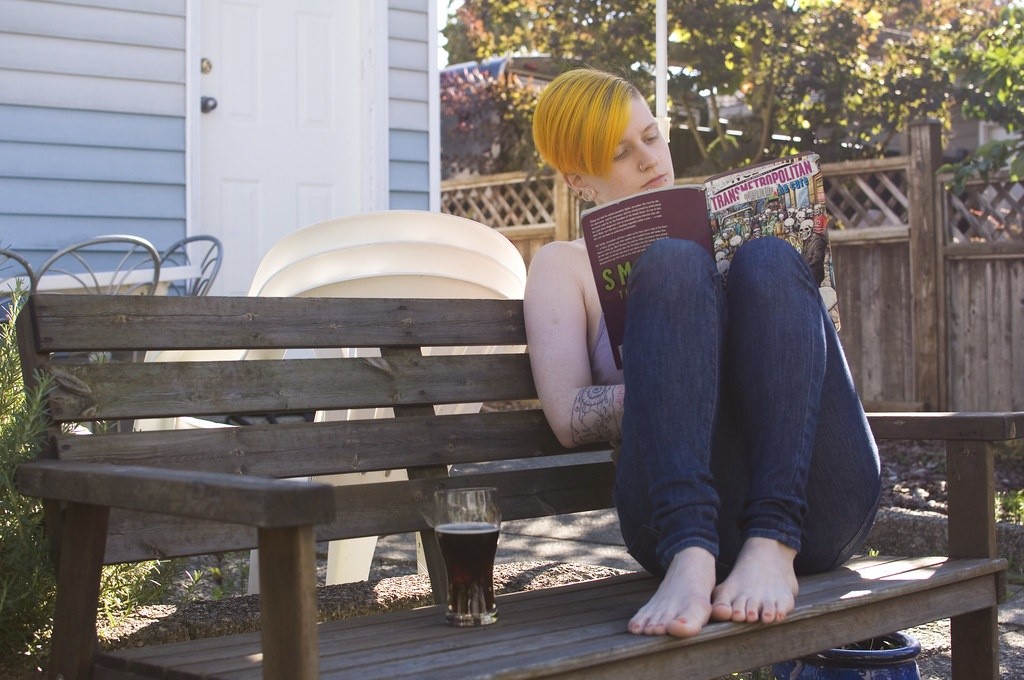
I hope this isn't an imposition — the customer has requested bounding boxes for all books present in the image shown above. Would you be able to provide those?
[580,150,841,371]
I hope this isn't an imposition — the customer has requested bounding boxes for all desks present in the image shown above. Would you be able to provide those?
[0,263,200,434]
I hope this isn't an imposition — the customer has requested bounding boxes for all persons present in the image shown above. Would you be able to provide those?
[523,69,884,638]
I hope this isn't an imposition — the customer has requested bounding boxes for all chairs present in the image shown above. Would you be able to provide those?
[0,208,528,598]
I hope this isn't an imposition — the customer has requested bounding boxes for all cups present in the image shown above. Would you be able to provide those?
[433,486,504,628]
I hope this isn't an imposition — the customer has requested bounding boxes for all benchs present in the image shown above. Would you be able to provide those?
[13,291,1024,680]
